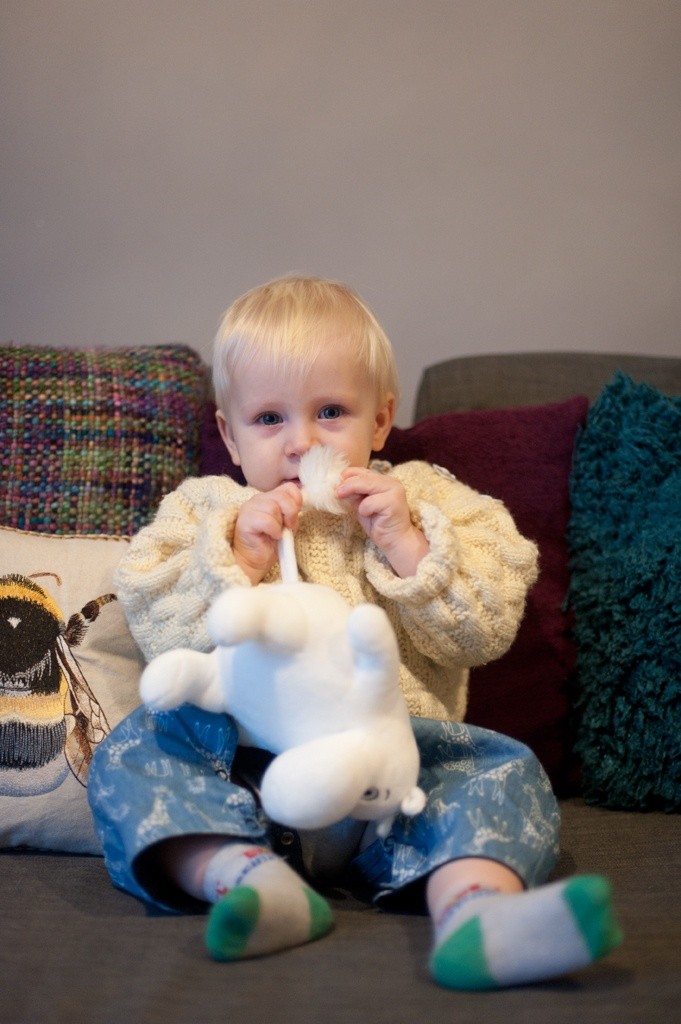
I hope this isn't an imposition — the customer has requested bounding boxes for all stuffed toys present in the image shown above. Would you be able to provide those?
[140,444,427,838]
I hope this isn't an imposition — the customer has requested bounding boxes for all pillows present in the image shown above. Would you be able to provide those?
[549,373,681,812]
[372,394,588,750]
[0,340,218,538]
[0,524,145,855]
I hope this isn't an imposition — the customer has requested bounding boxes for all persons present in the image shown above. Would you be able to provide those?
[85,277,623,990]
